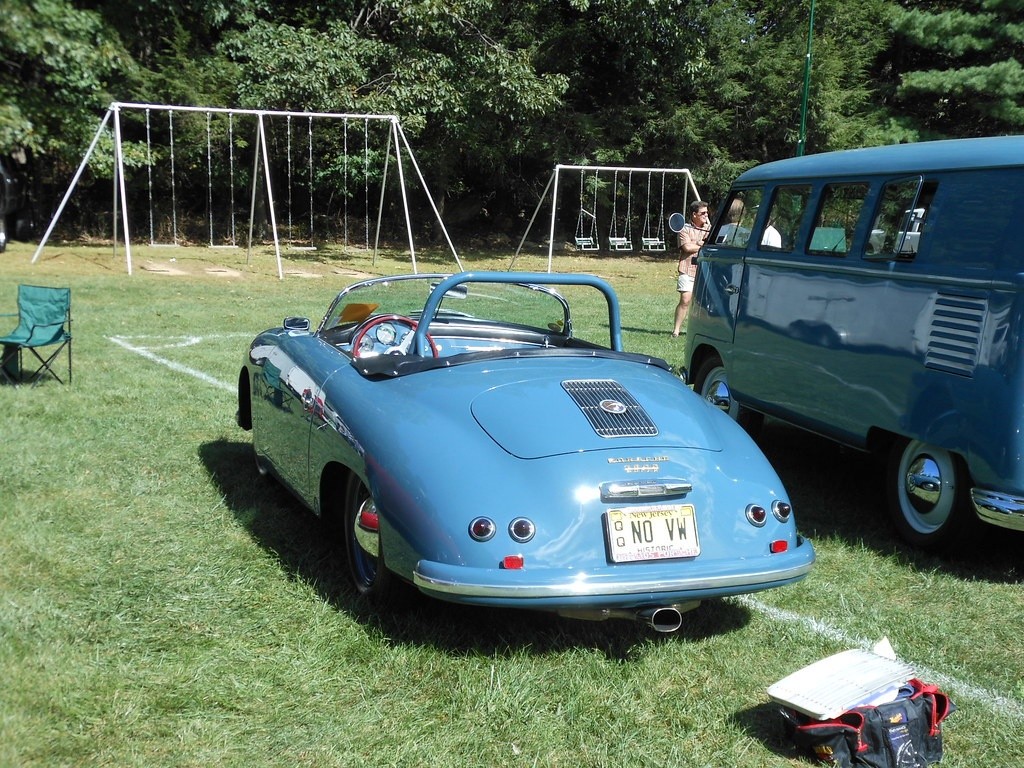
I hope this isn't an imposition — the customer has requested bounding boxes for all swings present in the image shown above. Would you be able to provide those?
[343,116,374,255]
[608,170,633,250]
[575,169,602,251]
[144,109,180,250]
[285,116,320,254]
[643,171,667,252]
[206,112,242,249]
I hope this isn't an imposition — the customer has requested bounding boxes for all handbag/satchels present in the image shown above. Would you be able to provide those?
[778,677,956,768]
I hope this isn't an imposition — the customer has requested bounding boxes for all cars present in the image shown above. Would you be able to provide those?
[234,268,816,635]
[864,206,927,257]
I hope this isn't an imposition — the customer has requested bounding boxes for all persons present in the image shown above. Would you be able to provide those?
[672,201,712,338]
[715,199,751,247]
[761,204,781,248]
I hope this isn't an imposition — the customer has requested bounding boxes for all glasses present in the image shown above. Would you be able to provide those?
[701,212,709,215]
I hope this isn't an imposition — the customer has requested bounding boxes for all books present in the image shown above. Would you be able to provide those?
[766,648,915,721]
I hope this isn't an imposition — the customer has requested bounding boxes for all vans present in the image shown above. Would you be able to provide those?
[679,132,1024,554]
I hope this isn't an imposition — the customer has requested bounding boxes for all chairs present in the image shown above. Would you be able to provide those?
[0,283,73,387]
